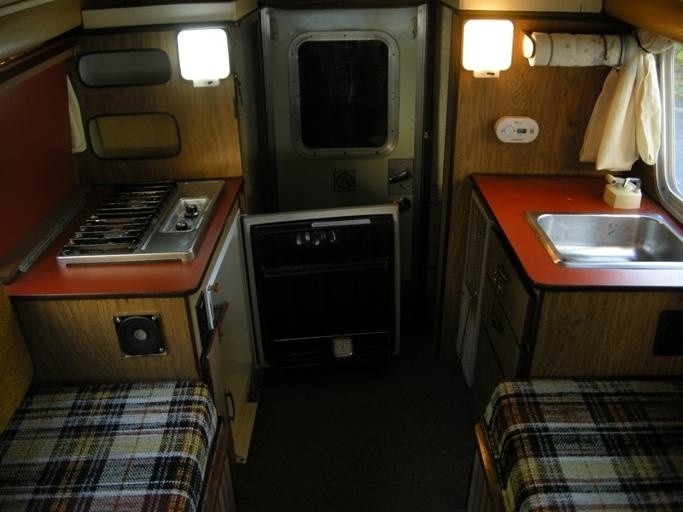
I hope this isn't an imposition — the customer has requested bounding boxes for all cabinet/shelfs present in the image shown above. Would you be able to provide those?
[474,226,682,418]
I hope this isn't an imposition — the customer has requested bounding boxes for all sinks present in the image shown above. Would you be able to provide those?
[527,209,683,268]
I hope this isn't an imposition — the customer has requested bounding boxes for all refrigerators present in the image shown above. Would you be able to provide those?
[3,174,401,461]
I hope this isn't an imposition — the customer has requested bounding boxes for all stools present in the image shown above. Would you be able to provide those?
[0,378,239,511]
[473,372,682,511]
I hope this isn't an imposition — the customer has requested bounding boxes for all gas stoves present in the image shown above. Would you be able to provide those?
[56,179,225,265]
